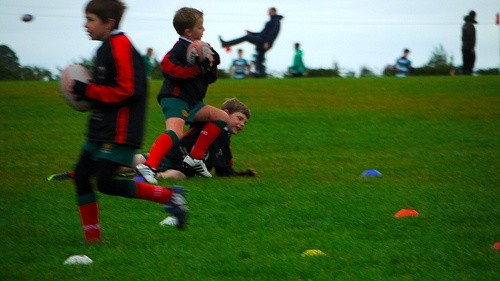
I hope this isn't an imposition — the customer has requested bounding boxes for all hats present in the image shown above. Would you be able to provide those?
[464,10,478,24]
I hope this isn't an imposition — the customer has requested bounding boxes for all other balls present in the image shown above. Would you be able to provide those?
[22,13,33,22]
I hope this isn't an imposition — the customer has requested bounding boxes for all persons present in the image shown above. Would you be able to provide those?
[287,43,308,76]
[460,9,479,74]
[216,7,284,77]
[47,97,258,189]
[228,48,252,76]
[136,7,230,185]
[65,0,189,248]
[394,49,411,76]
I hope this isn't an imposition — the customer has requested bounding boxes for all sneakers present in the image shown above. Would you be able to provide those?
[163,185,189,231]
[183,155,213,179]
[136,163,158,185]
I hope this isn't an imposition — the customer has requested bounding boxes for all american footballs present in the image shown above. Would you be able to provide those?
[188,40,215,73]
[61,64,97,113]
